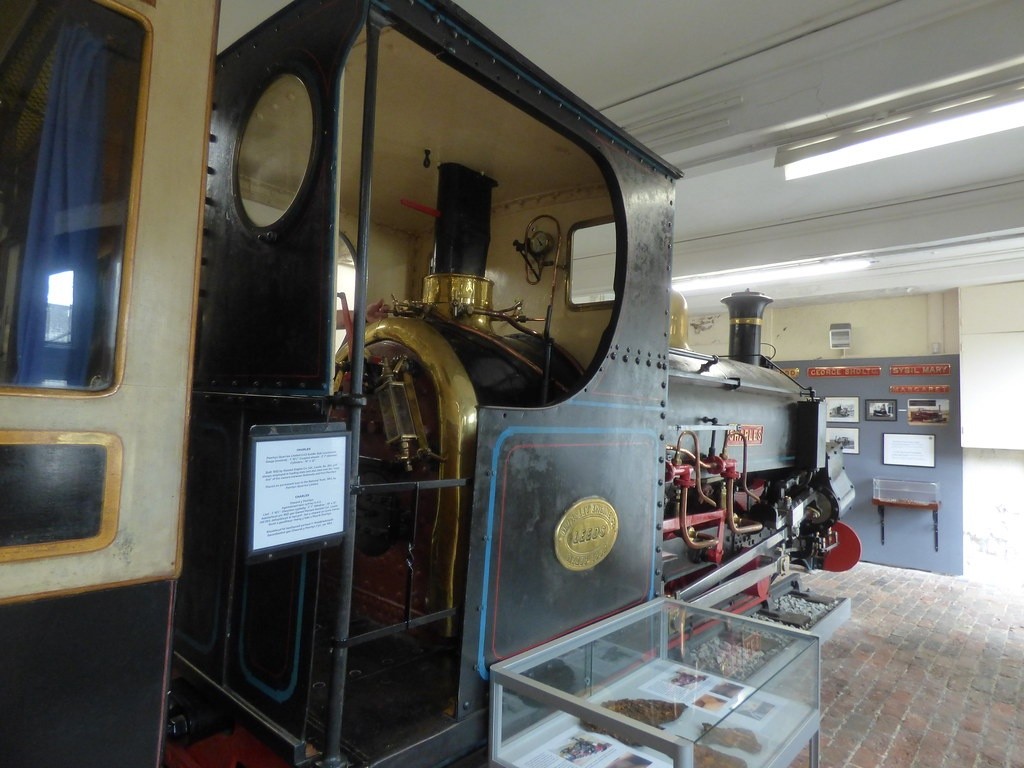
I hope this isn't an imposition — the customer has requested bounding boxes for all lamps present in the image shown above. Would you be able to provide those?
[774,81,1024,182]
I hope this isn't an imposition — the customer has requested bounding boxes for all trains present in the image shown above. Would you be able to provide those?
[0,0,857,768]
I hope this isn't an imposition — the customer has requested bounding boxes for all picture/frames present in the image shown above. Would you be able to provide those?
[827,427,860,455]
[864,399,897,421]
[882,432,936,469]
[825,396,860,423]
